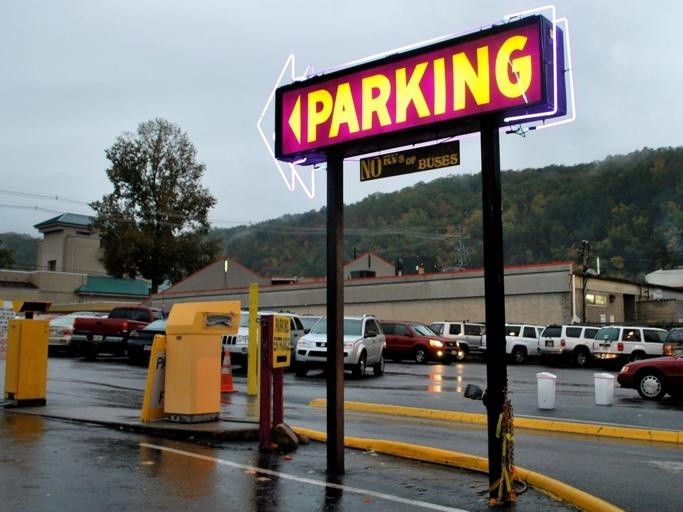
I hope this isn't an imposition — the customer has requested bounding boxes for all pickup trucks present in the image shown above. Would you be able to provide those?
[70,304,165,359]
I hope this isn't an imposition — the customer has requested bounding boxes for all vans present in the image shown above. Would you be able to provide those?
[593,324,668,363]
[479,323,545,362]
[431,321,484,356]
[381,319,465,365]
[538,324,601,366]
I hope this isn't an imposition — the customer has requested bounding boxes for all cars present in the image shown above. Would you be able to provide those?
[127,318,166,364]
[616,355,682,401]
[663,328,682,357]
[47,311,105,346]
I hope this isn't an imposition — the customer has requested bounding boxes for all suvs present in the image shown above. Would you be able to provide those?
[221,311,387,376]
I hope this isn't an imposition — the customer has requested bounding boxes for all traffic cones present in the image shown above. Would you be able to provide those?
[222,349,239,393]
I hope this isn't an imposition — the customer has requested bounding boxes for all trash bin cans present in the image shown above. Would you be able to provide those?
[535,372,615,409]
